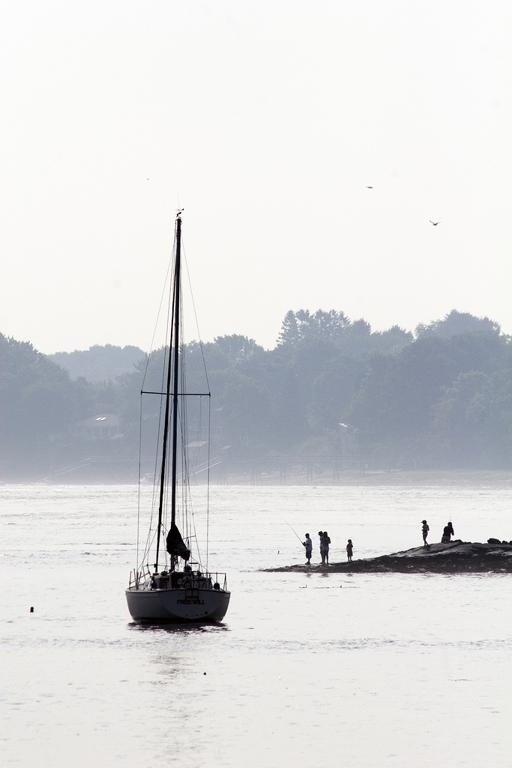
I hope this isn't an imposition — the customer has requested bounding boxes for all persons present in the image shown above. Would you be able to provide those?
[319,531,324,564]
[421,520,429,546]
[441,521,454,543]
[144,565,220,591]
[321,532,331,565]
[302,533,312,565]
[346,539,353,563]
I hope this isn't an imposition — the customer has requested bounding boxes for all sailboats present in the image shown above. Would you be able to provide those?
[124,205,231,629]
